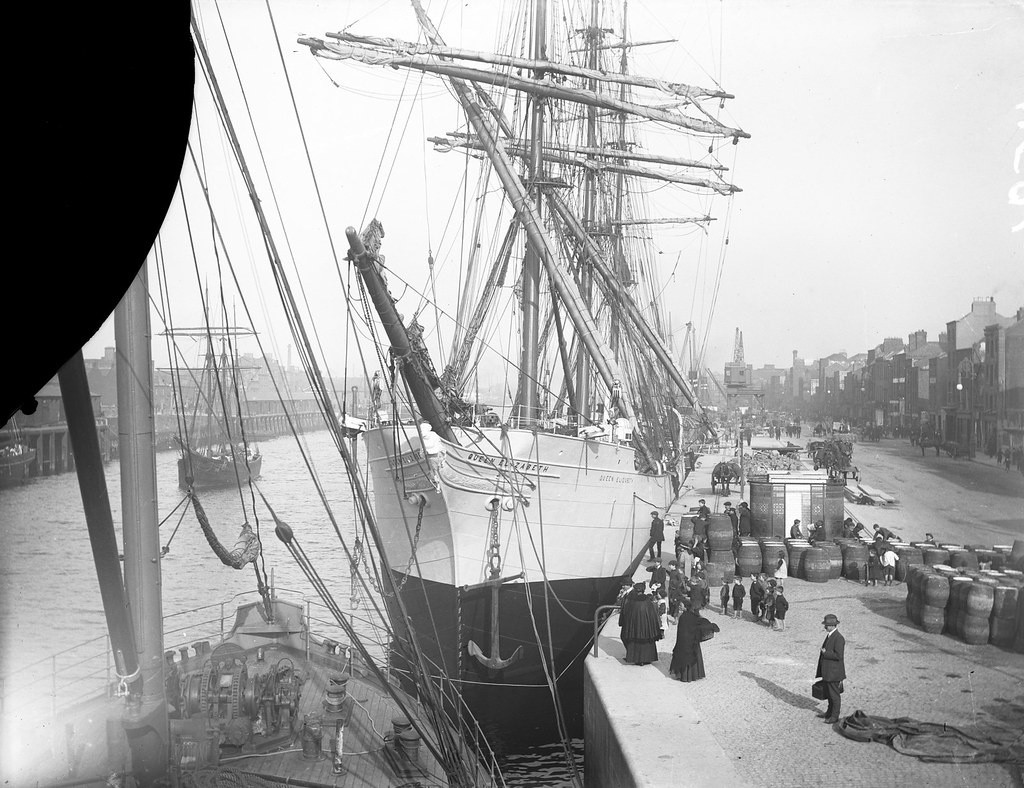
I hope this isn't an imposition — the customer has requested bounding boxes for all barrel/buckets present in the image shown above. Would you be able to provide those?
[681,512,736,585]
[738,536,870,582]
[885,538,1024,654]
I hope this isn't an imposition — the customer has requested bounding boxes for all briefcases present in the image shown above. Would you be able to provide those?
[812,680,843,700]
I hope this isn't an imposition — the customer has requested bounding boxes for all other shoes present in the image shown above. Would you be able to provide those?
[818,712,830,718]
[825,717,838,723]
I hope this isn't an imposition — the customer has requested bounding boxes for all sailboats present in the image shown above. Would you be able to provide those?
[158,303,263,492]
[136,0,754,788]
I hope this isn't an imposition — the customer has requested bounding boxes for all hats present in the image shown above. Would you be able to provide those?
[696,572,705,579]
[653,557,662,563]
[822,614,840,625]
[633,583,646,591]
[620,577,634,584]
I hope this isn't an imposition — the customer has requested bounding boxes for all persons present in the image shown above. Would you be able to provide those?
[815,614,845,723]
[722,411,1024,474]
[618,500,936,682]
